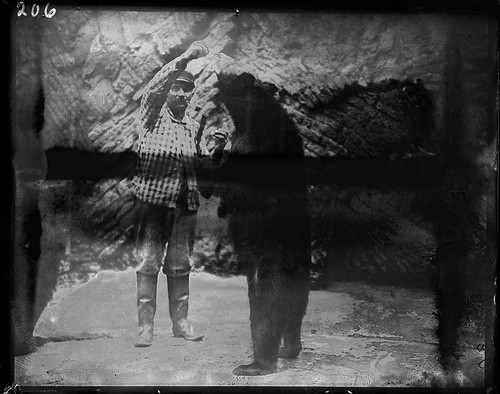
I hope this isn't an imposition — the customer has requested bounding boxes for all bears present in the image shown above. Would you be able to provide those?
[209,72,312,375]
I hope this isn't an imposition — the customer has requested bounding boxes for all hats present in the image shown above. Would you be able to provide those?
[174,70,193,82]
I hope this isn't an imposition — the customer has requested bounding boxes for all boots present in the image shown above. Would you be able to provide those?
[135,271,157,347]
[167,272,203,341]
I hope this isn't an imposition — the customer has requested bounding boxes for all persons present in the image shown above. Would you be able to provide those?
[134,39,231,348]
